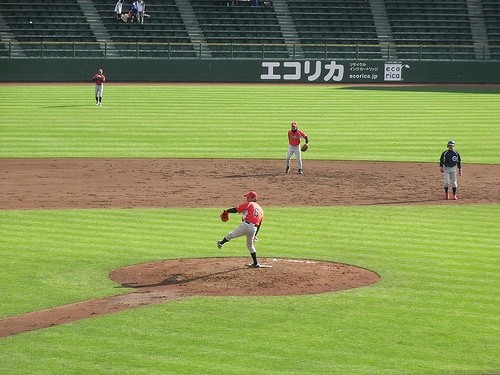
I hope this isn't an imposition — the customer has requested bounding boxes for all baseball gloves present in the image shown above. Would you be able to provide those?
[301,144,308,152]
[97,76,101,81]
[220,210,229,222]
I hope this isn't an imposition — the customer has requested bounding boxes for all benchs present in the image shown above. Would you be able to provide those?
[0,0,500,62]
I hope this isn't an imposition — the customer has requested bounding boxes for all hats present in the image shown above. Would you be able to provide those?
[447,140,455,146]
[291,122,297,127]
[244,191,257,201]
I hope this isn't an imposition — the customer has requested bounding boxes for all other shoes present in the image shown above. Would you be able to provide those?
[445,192,449,199]
[217,240,223,249]
[249,263,262,268]
[452,194,457,200]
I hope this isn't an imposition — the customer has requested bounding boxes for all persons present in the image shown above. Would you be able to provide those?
[93,68,106,107]
[114,0,147,26]
[217,191,264,269]
[439,141,462,200]
[285,121,308,175]
[252,0,259,6]
